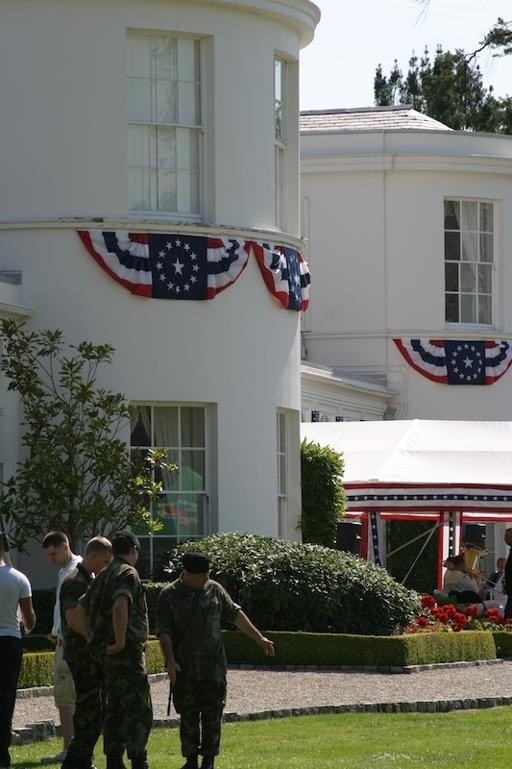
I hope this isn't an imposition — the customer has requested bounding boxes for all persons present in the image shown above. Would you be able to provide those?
[156,551,275,769]
[443,527,512,621]
[41,530,153,769]
[0,532,36,769]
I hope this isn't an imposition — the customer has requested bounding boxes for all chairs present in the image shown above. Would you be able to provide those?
[448,589,487,612]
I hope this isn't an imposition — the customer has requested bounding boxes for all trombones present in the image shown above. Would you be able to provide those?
[440,561,504,593]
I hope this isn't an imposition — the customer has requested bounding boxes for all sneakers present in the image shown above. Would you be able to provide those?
[180,755,214,769]
[41,752,68,763]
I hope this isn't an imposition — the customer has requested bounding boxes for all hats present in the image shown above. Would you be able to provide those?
[115,530,141,551]
[182,552,209,574]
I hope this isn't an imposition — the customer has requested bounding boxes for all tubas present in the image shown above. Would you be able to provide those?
[461,543,488,571]
